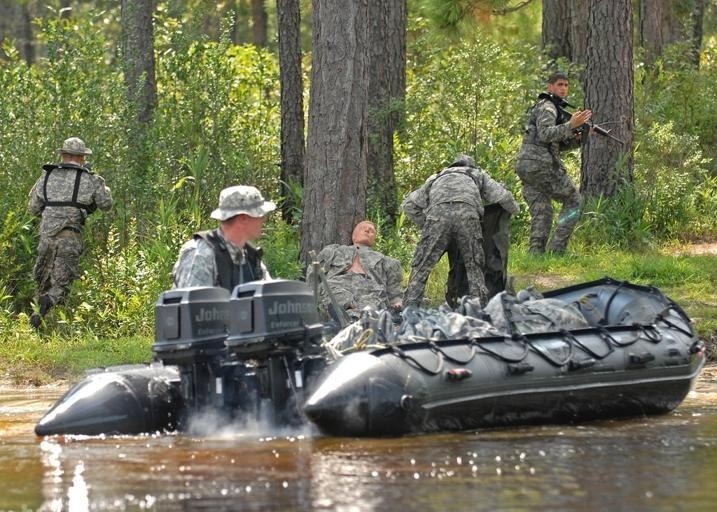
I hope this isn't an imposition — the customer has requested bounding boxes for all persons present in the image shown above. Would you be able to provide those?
[515,72,597,257]
[28,137,114,328]
[172,185,277,292]
[402,155,520,308]
[306,221,404,323]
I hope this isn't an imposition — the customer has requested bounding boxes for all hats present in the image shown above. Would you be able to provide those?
[449,155,478,167]
[57,136,94,155]
[210,182,278,220]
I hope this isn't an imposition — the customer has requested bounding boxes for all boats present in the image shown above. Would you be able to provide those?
[32,274,707,441]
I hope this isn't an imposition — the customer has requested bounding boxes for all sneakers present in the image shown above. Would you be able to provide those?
[31,297,50,328]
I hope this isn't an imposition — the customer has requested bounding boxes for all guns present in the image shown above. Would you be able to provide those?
[550,93,626,145]
